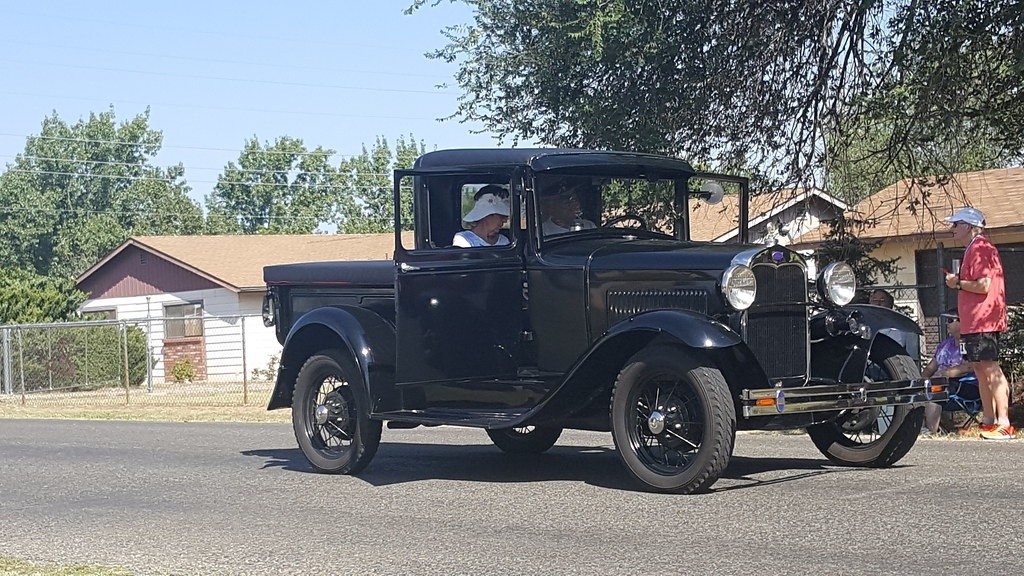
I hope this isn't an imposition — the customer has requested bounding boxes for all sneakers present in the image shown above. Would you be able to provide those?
[958,423,1017,439]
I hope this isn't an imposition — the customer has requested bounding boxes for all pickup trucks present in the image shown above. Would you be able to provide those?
[259,143,962,506]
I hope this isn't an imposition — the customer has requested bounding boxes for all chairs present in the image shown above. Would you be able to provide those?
[937,375,984,437]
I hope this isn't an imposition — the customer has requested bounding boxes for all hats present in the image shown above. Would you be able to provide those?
[944,207,986,228]
[940,306,960,318]
[462,193,511,223]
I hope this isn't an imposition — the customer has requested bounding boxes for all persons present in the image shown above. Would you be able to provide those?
[453,185,512,247]
[524,177,596,257]
[870,288,894,309]
[943,207,1016,439]
[920,306,981,439]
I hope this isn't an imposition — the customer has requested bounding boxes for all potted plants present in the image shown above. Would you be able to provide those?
[172,359,194,385]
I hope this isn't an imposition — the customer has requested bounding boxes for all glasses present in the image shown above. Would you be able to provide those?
[954,222,968,228]
[947,317,960,323]
[870,297,890,303]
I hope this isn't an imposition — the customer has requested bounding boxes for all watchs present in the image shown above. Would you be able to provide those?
[956,280,961,290]
